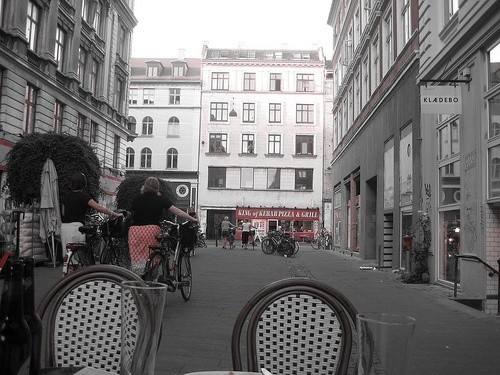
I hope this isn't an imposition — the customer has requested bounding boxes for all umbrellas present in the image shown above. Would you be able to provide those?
[38,159,64,268]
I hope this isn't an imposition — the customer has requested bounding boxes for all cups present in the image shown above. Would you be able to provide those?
[120,279,169,375]
[355,311,417,375]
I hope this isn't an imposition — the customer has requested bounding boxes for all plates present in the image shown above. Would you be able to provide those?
[184,370,275,375]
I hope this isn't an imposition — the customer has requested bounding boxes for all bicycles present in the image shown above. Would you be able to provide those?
[249,228,257,250]
[168,225,208,255]
[261,227,299,257]
[60,211,133,277]
[310,230,332,250]
[143,219,194,301]
[228,227,238,250]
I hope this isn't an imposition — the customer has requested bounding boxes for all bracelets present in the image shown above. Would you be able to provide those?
[110,211,113,217]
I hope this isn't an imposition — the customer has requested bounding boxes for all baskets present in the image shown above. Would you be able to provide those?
[167,226,198,248]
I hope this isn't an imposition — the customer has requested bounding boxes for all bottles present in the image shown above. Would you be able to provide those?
[17,257,43,375]
[0,256,31,375]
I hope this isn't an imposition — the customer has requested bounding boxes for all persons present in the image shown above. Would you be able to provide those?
[128,176,200,282]
[187,208,200,234]
[220,216,236,248]
[61,171,123,279]
[241,219,257,249]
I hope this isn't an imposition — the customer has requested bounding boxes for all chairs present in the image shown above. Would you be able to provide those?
[230,277,375,375]
[29,264,157,375]
